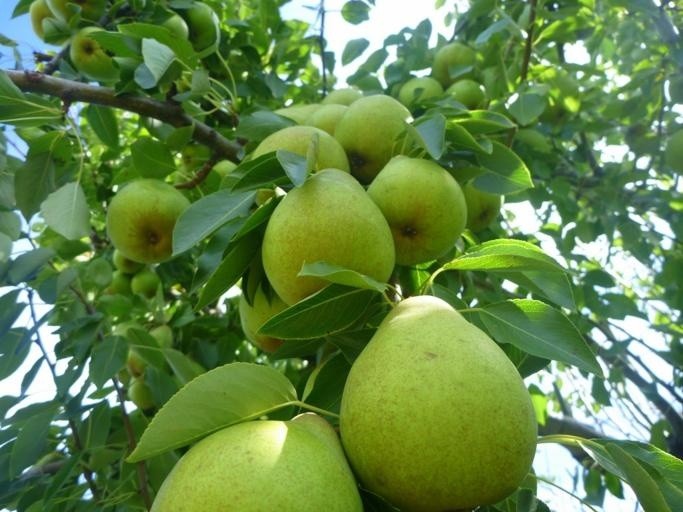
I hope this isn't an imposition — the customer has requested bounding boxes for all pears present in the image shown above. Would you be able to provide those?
[29,1,537,512]
